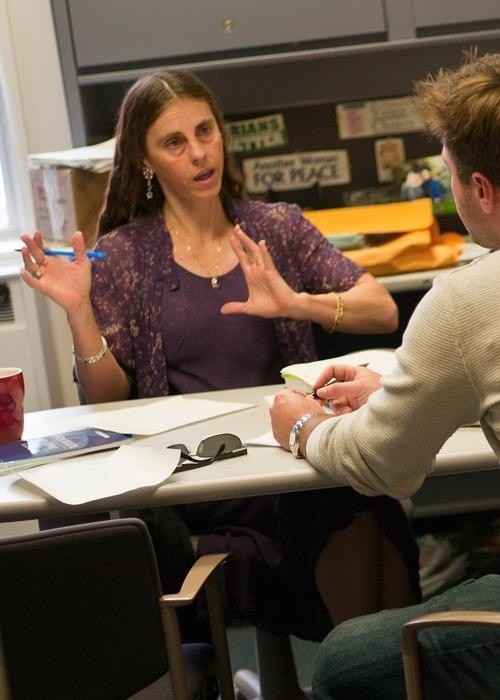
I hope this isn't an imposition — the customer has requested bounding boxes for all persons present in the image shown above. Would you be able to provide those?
[268,47,500,699]
[16,69,422,640]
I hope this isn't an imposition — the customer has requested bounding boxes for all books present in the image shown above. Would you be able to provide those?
[0,428,133,477]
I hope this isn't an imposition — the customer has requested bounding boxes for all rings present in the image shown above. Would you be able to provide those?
[36,268,42,278]
[253,260,264,267]
[39,255,47,266]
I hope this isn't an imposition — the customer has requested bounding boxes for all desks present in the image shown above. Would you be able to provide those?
[0,369,499,700]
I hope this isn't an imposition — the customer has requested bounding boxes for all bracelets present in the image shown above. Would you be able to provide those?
[322,292,344,333]
[70,336,108,365]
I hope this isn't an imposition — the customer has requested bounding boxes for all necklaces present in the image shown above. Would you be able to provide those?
[164,213,230,291]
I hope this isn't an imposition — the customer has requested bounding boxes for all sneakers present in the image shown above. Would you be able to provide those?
[417,531,485,598]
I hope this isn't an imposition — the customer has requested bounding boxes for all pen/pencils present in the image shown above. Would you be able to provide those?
[15,250,108,261]
[303,363,369,401]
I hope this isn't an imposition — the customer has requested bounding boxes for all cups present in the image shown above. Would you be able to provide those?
[0,366,26,447]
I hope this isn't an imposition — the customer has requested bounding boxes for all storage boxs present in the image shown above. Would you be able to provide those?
[28,160,112,247]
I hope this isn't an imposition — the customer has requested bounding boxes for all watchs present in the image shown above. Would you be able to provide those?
[288,411,324,458]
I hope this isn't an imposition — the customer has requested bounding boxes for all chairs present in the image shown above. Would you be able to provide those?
[399,608,500,700]
[1,515,238,700]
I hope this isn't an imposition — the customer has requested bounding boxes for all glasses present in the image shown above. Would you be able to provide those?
[166,432,248,474]
